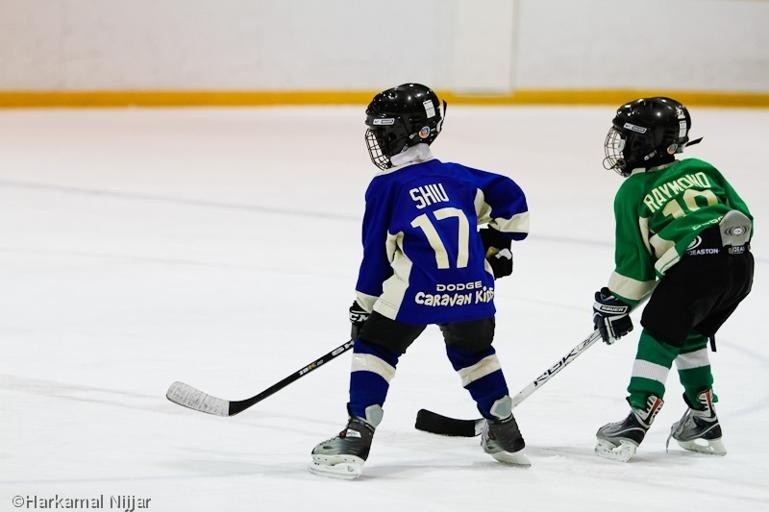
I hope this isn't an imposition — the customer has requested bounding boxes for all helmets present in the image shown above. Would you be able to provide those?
[364,84,447,172]
[602,97,703,176]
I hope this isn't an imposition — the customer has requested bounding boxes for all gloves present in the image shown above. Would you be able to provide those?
[592,287,633,345]
[478,228,513,280]
[349,300,370,343]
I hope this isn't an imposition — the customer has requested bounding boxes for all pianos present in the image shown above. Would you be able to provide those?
[166,247,513,417]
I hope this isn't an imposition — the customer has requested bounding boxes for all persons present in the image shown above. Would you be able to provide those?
[310,80,530,465]
[592,97,754,445]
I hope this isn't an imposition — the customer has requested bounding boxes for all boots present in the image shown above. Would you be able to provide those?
[596,393,664,447]
[311,402,375,461]
[671,386,722,441]
[477,402,525,453]
